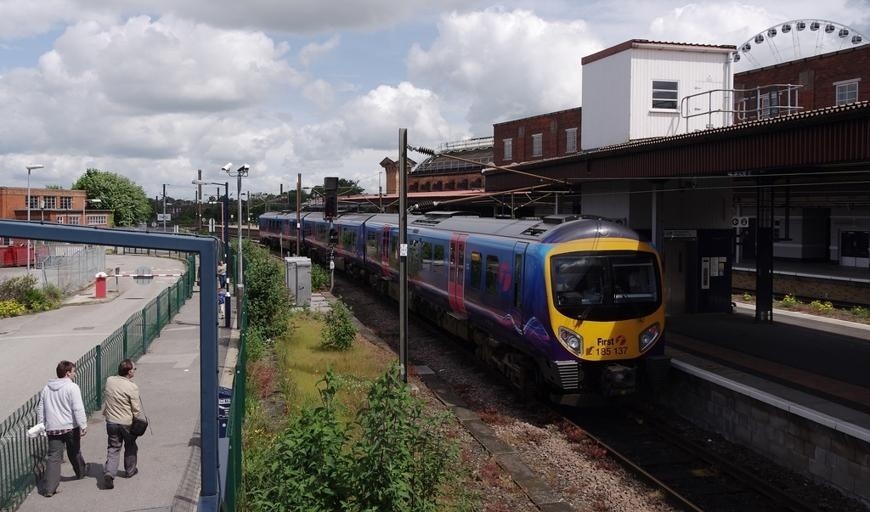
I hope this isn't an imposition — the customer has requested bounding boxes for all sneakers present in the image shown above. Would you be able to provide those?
[77,463,90,479]
[43,483,59,497]
[126,468,138,477]
[104,476,114,489]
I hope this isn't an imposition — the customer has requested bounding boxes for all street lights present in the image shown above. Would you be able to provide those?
[195,178,229,276]
[224,162,250,284]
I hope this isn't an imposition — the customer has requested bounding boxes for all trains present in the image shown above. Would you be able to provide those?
[257,208,669,414]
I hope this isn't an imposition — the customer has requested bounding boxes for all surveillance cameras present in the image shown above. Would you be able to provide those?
[222,163,232,170]
[244,164,249,171]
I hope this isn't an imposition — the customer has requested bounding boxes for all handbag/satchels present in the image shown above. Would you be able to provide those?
[131,417,148,436]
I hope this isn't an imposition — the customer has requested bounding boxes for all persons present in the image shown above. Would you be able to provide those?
[217,294,226,320]
[38,359,89,496]
[102,358,151,489]
[217,260,227,291]
